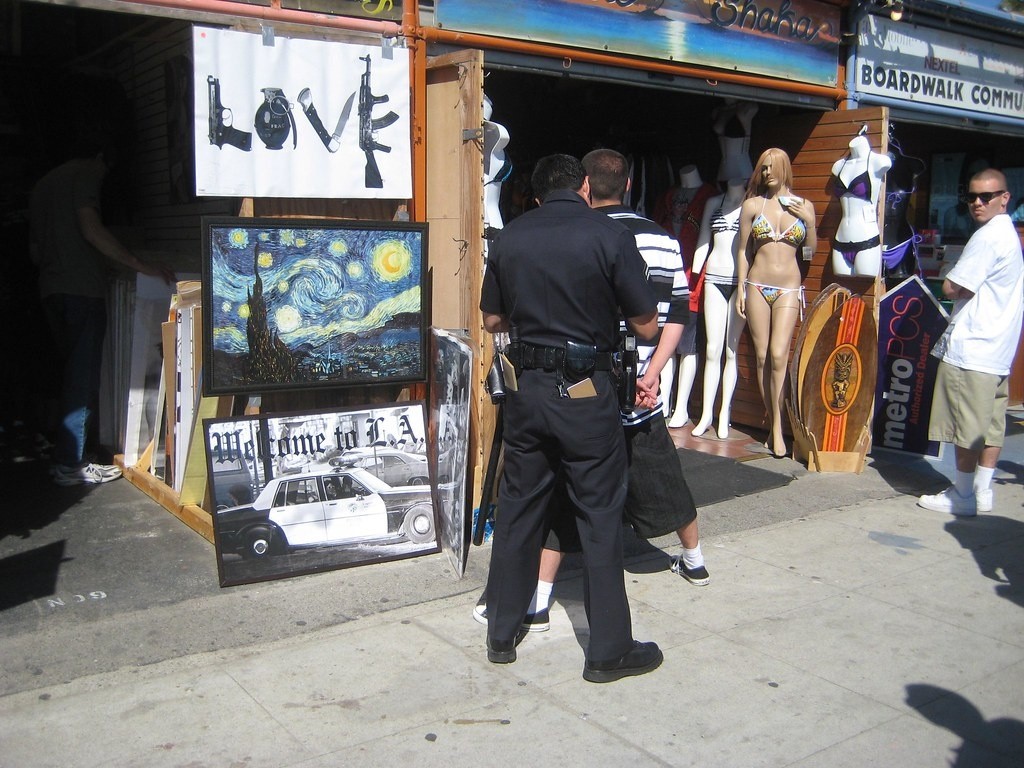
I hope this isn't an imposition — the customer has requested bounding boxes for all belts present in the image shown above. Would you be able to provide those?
[525,349,614,372]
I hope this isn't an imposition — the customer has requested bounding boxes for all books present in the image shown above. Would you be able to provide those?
[567,378,598,398]
[501,355,519,391]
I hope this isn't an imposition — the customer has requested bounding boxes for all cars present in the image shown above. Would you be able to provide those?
[334,450,429,490]
[219,469,439,558]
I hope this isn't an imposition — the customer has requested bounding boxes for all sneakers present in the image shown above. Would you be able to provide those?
[670,553,710,587]
[976,489,992,511]
[474,604,550,632]
[50,458,122,487]
[918,484,977,516]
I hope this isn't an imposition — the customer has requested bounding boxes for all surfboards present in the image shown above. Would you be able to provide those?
[789,283,879,452]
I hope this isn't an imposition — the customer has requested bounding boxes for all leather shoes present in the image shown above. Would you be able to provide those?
[486,628,522,663]
[583,640,664,683]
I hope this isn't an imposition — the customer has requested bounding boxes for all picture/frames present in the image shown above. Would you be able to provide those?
[200,213,433,399]
[201,400,444,590]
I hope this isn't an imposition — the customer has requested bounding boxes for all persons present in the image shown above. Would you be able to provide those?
[882,136,926,293]
[30,134,178,487]
[832,136,892,277]
[481,93,512,349]
[480,154,710,683]
[653,96,817,456]
[918,168,1024,516]
[324,475,365,500]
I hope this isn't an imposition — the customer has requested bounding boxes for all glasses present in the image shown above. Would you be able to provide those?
[965,190,1004,203]
